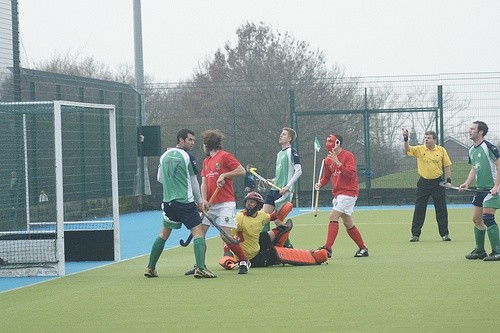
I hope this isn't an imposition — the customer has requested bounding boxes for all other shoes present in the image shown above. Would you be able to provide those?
[144,268,158,277]
[193,267,217,279]
[354,247,369,257]
[311,245,332,258]
[483,251,500,261]
[237,259,251,274]
[410,236,419,242]
[465,248,488,259]
[442,235,451,241]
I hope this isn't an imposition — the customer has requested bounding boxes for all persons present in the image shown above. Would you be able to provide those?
[458,120,500,262]
[243,164,256,193]
[219,190,328,269]
[315,134,369,258]
[261,128,302,248]
[184,128,251,276]
[402,128,451,242]
[143,128,217,279]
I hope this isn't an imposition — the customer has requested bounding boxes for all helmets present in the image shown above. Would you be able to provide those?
[244,192,264,216]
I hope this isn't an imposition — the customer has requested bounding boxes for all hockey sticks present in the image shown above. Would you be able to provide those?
[439,180,492,194]
[179,181,223,248]
[313,159,325,216]
[196,203,242,245]
[248,166,287,196]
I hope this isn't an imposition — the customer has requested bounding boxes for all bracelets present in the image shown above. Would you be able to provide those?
[404,136,408,142]
[447,177,451,183]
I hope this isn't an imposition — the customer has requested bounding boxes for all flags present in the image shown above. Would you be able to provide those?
[314,138,321,152]
